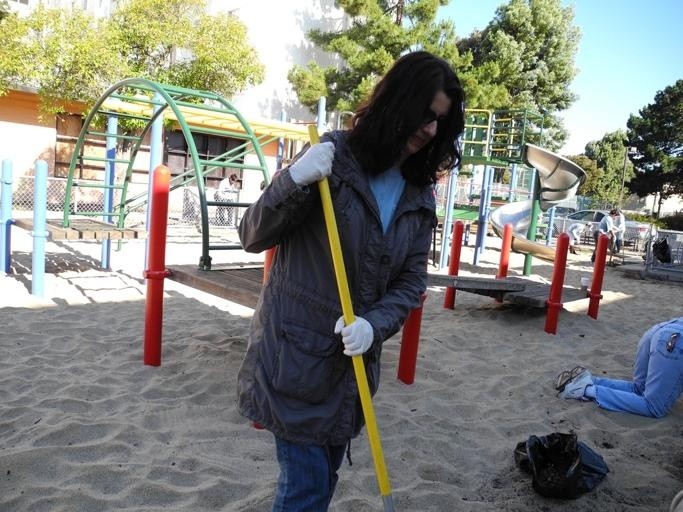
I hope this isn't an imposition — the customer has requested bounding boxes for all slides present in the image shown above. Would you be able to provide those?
[488,143,587,267]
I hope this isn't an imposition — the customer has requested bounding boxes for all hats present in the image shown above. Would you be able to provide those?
[586,222,595,232]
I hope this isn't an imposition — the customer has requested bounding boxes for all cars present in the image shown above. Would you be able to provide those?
[542,206,658,246]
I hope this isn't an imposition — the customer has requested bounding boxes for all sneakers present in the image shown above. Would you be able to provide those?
[553,365,594,401]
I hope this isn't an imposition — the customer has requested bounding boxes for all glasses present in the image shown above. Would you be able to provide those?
[666,333,680,353]
[422,106,448,130]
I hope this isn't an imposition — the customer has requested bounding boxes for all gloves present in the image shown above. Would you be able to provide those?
[288,141,335,190]
[333,314,375,357]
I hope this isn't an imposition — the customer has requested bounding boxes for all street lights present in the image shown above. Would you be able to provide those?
[618,147,637,209]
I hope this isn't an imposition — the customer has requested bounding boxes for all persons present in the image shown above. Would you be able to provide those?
[566,223,593,255]
[552,315,683,419]
[232,52,466,512]
[614,208,626,253]
[214,173,237,225]
[590,209,620,266]
